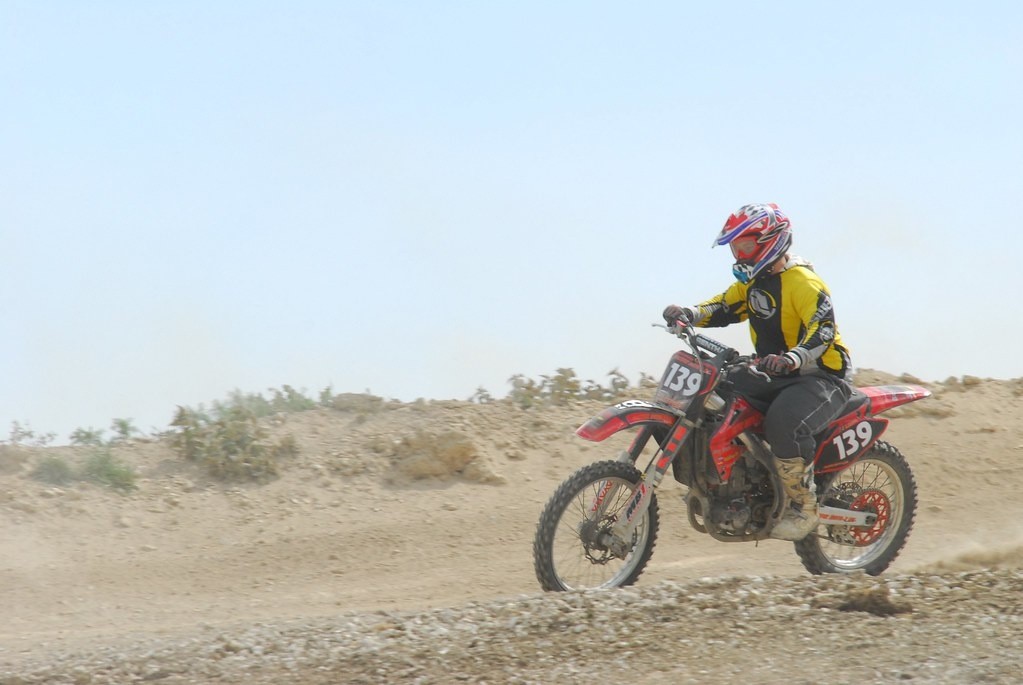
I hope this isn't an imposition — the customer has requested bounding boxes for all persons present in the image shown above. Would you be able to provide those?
[663,203,853,543]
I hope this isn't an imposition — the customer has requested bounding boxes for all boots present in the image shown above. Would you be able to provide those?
[769,453,819,541]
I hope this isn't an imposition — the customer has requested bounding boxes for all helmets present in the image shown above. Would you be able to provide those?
[710,203,792,285]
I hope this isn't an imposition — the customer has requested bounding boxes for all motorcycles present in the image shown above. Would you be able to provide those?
[532,308,932,594]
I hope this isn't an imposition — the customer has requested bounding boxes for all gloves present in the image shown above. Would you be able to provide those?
[663,305,694,328]
[759,353,795,379]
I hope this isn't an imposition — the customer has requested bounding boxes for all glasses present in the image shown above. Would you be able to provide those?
[729,235,763,261]
